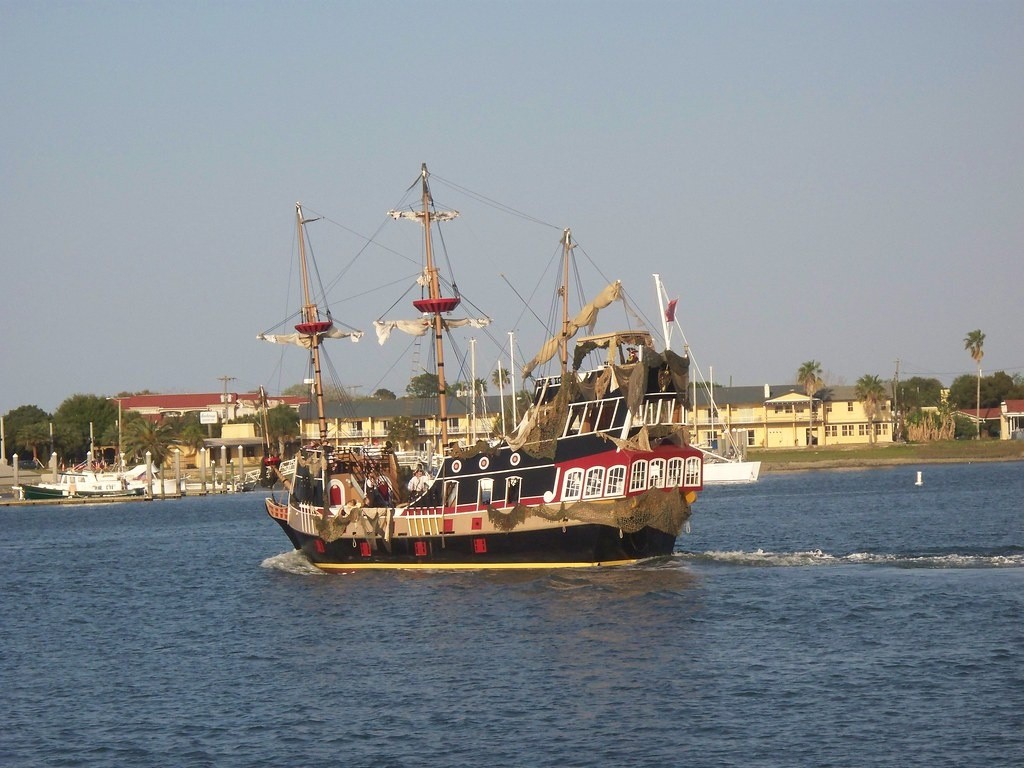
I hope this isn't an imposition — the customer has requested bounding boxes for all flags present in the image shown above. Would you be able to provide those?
[664,298,678,323]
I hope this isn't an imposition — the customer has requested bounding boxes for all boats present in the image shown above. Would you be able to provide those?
[13,460,190,497]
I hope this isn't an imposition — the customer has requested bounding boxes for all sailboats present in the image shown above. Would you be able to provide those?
[254,163,709,576]
[651,270,764,486]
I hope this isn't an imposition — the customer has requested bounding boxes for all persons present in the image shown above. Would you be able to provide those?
[407,468,432,500]
[366,472,376,508]
